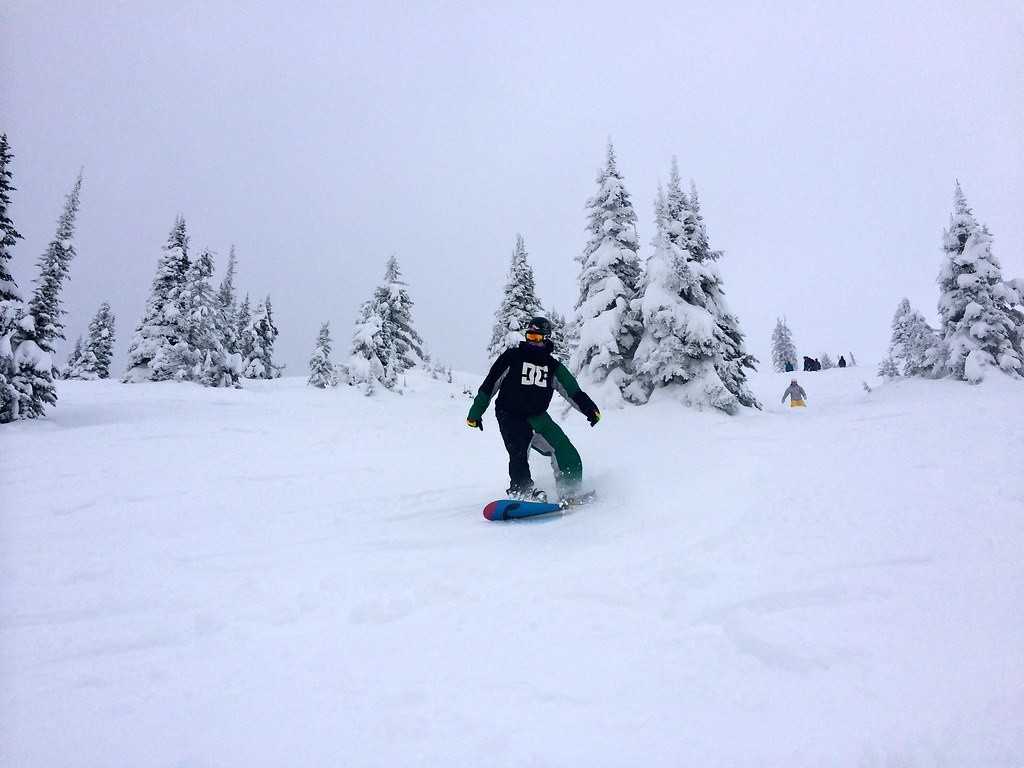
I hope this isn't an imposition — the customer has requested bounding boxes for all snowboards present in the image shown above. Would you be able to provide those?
[482,489,597,521]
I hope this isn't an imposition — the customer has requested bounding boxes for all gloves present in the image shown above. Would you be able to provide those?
[467,417,483,431]
[586,406,601,427]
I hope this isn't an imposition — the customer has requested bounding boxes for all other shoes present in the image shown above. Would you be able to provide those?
[558,486,595,504]
[510,484,546,502]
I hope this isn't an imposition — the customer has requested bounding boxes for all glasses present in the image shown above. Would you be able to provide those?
[525,330,551,342]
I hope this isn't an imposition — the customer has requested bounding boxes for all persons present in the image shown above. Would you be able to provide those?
[839,356,846,368]
[803,356,821,371]
[467,316,601,505]
[782,380,807,407]
[786,361,793,372]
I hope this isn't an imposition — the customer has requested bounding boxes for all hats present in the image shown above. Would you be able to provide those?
[527,317,551,334]
